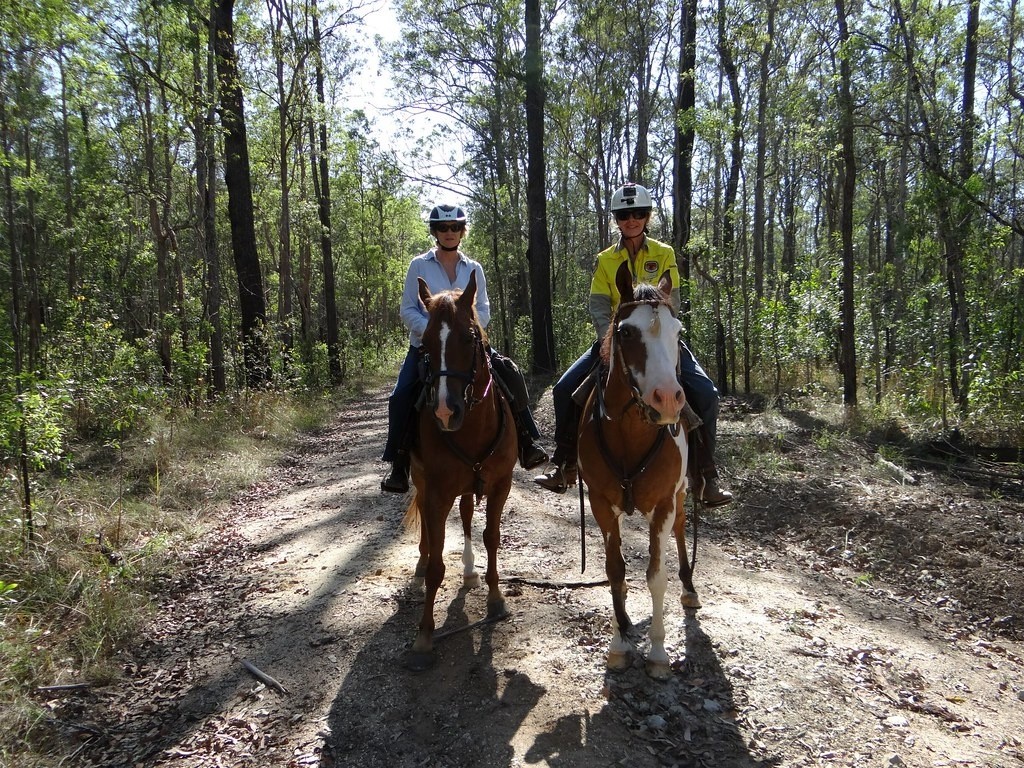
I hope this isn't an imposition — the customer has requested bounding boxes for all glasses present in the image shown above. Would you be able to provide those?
[613,209,648,220]
[431,224,462,232]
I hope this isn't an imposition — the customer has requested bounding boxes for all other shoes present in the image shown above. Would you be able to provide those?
[518,444,547,467]
[387,461,409,491]
[534,463,577,486]
[692,480,733,504]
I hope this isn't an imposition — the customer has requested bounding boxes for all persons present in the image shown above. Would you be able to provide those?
[534,183,734,508]
[382,204,549,493]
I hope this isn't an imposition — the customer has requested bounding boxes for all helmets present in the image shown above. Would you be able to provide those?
[611,183,652,213]
[429,206,466,223]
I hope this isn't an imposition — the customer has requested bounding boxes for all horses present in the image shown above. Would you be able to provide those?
[393,267,518,677]
[575,257,702,682]
